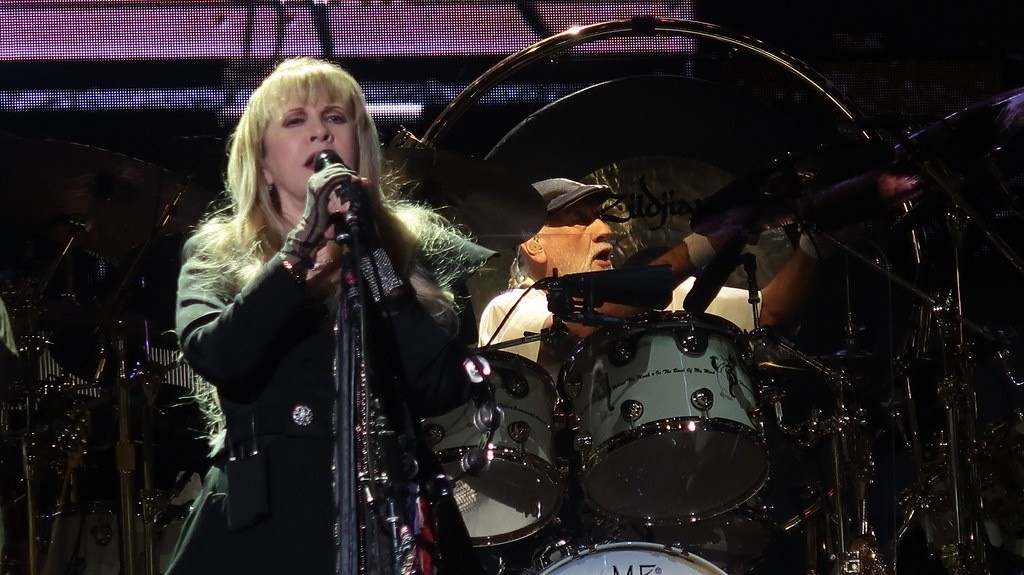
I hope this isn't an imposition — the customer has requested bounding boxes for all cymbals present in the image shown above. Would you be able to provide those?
[2,136,217,255]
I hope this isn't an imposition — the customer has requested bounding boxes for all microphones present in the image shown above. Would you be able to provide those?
[312,148,361,233]
[682,213,760,314]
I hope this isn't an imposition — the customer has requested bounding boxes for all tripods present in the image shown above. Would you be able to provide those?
[886,361,961,575]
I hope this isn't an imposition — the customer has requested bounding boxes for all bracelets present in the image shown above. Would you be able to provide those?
[798,230,823,257]
[682,233,718,270]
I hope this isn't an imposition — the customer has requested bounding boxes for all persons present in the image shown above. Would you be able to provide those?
[617,173,925,335]
[164,55,503,575]
[478,177,757,362]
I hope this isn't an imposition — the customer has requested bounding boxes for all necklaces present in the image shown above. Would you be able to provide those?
[308,256,341,271]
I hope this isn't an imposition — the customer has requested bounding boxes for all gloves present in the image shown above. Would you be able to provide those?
[798,172,880,262]
[682,206,775,269]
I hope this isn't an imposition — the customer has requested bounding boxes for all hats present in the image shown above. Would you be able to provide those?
[525,177,612,217]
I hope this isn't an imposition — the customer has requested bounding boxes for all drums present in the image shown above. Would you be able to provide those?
[424,348,563,550]
[521,538,726,575]
[564,308,770,526]
